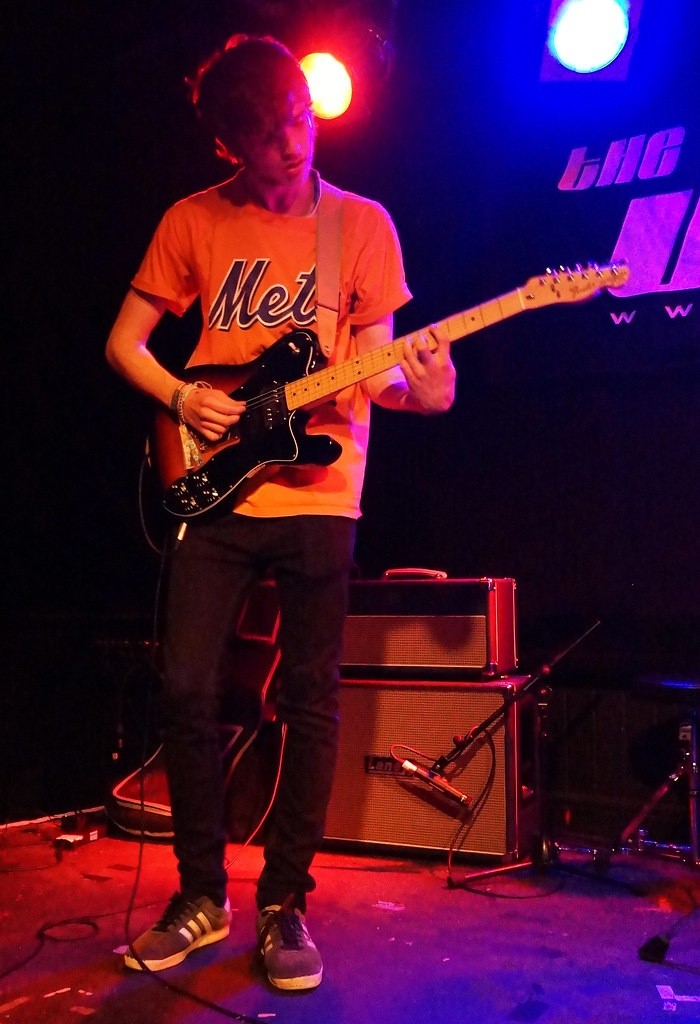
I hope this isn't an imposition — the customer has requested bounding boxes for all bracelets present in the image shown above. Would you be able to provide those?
[179,381,212,470]
[170,382,187,412]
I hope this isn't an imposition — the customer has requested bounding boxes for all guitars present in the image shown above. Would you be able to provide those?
[144,260,632,519]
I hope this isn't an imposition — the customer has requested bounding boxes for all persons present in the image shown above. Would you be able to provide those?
[106,32,457,991]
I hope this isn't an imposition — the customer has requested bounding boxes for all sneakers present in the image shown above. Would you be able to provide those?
[124,890,232,972]
[255,902,324,992]
